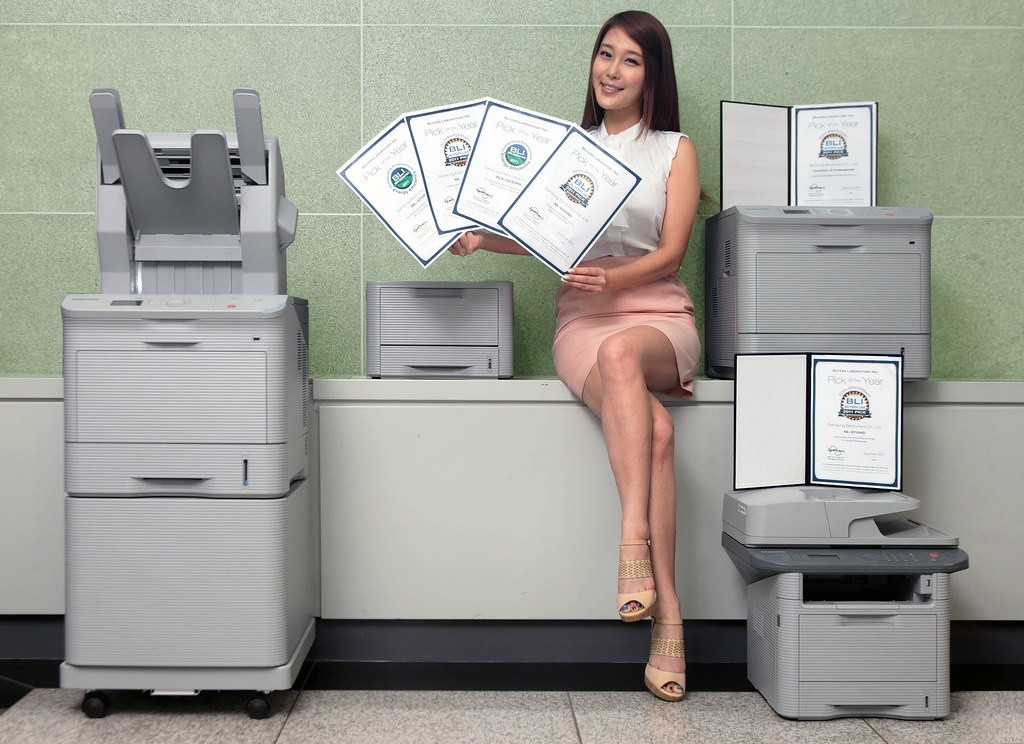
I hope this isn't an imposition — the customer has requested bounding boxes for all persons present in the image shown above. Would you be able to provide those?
[450,11,702,701]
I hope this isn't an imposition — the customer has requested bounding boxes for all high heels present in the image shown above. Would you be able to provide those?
[644,618,686,701]
[617,540,657,622]
